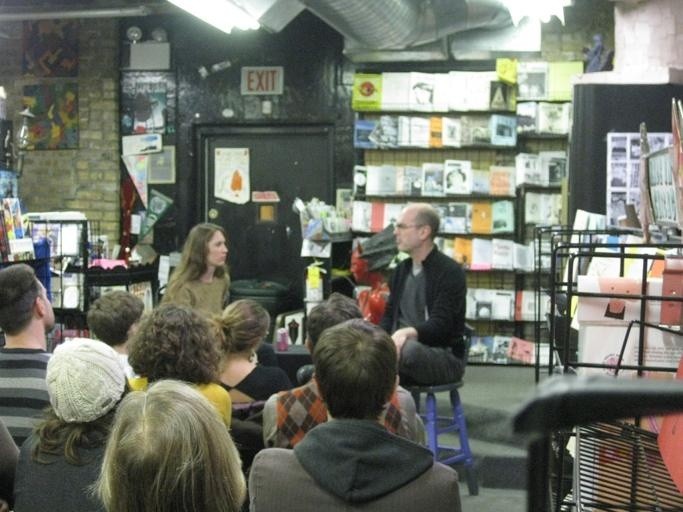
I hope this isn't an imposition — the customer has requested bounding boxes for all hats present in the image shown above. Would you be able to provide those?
[47,336,128,424]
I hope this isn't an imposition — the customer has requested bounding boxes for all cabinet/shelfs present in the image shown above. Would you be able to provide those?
[355,62,570,368]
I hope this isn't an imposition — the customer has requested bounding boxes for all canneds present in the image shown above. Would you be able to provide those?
[277,328,288,352]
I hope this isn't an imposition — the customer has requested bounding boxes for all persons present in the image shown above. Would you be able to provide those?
[257,290,431,451]
[242,318,462,511]
[0,260,75,444]
[80,380,249,511]
[208,297,297,403]
[8,336,133,511]
[125,299,237,429]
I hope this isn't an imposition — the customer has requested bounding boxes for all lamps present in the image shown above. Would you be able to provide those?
[126,25,171,72]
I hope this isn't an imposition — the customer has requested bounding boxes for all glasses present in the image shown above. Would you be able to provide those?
[397,223,416,229]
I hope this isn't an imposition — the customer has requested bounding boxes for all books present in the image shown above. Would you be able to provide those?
[2,173,109,266]
[354,150,568,196]
[351,64,584,109]
[335,188,561,233]
[465,288,550,321]
[353,102,575,149]
[434,237,530,271]
[465,324,555,365]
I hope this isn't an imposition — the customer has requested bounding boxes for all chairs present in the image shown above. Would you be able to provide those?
[404,323,479,497]
